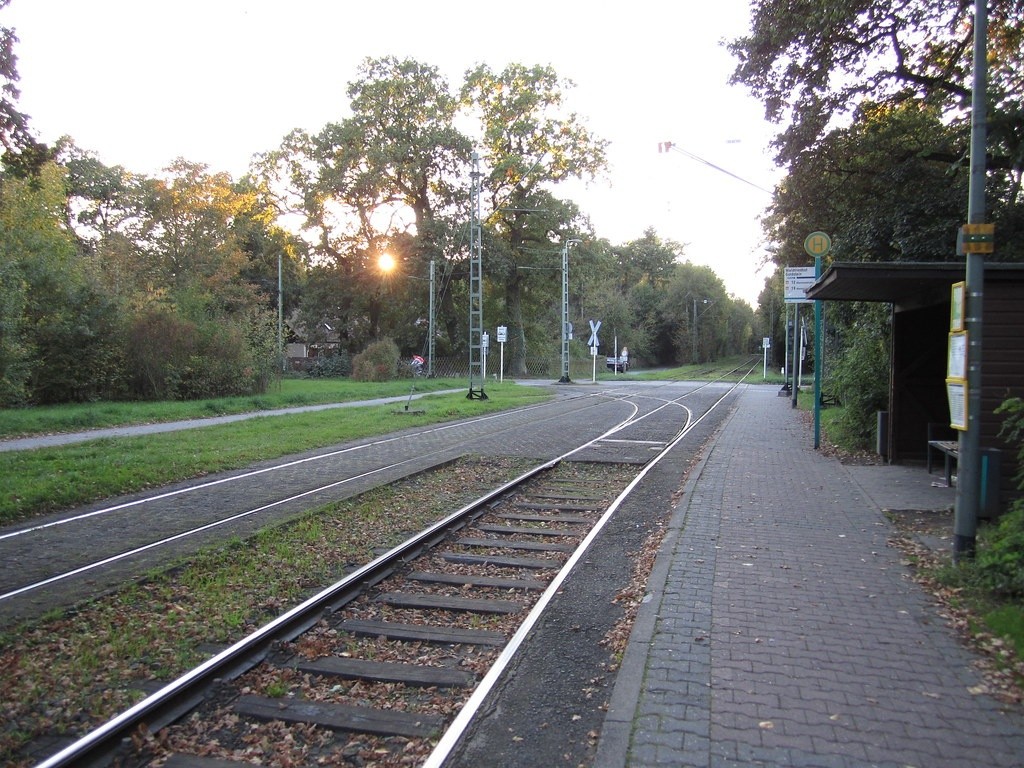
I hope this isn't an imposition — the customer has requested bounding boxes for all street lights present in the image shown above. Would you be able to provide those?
[559,239,582,384]
[693,300,707,365]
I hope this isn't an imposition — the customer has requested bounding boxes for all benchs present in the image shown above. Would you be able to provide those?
[927,420,959,486]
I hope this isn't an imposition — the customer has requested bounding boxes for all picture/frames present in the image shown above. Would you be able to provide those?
[950,281,965,331]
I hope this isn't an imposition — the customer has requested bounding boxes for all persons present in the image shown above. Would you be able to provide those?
[411,351,425,374]
